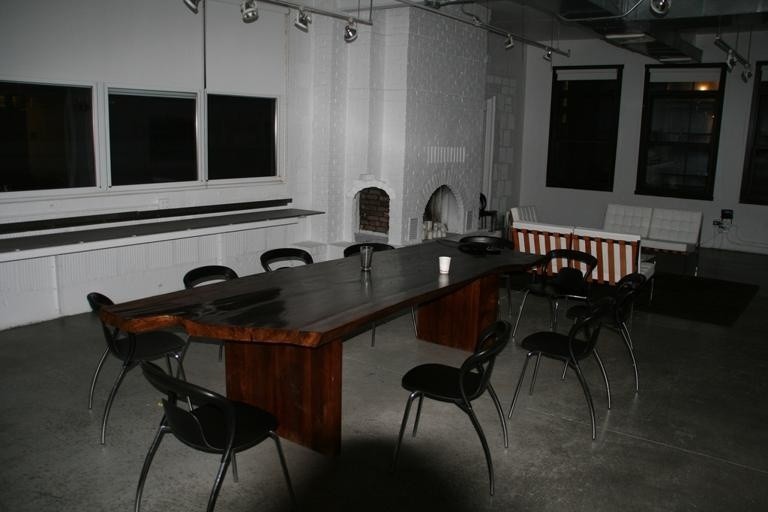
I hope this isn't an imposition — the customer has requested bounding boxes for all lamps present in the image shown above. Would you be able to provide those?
[494,31,571,69]
[184,0,373,45]
[709,23,757,88]
[649,0,672,12]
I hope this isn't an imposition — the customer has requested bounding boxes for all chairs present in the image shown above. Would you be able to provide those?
[87,234,647,507]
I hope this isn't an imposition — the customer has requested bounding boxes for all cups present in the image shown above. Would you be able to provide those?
[360,245,375,270]
[360,268,372,288]
[438,274,450,287]
[438,256,452,275]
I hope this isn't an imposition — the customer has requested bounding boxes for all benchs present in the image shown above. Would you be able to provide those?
[594,204,704,284]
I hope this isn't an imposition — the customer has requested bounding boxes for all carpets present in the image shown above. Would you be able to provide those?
[641,269,760,326]
